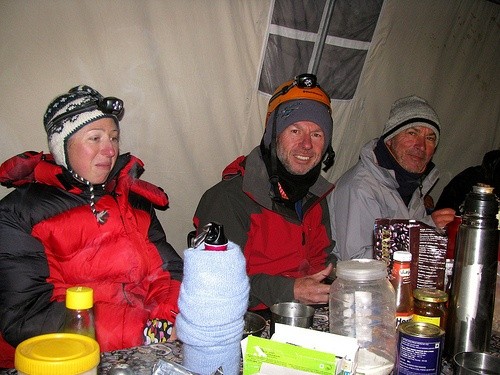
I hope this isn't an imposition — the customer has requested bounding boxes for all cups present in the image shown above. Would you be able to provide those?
[269,302,315,340]
[453,352,500,375]
[243,311,267,339]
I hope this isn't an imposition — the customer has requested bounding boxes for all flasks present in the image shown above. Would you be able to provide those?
[441,182,498,359]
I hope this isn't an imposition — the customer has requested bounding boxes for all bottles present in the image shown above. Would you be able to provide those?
[63,286,95,339]
[175,220,251,375]
[328,258,397,375]
[391,250,414,329]
[14,333,100,375]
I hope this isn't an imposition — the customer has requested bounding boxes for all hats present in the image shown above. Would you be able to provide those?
[43,84,120,224]
[381,95,440,148]
[263,79,333,157]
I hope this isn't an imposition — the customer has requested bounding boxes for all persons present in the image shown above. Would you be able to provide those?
[434,150,500,218]
[327,94,457,260]
[192,76,337,311]
[1,82,184,370]
